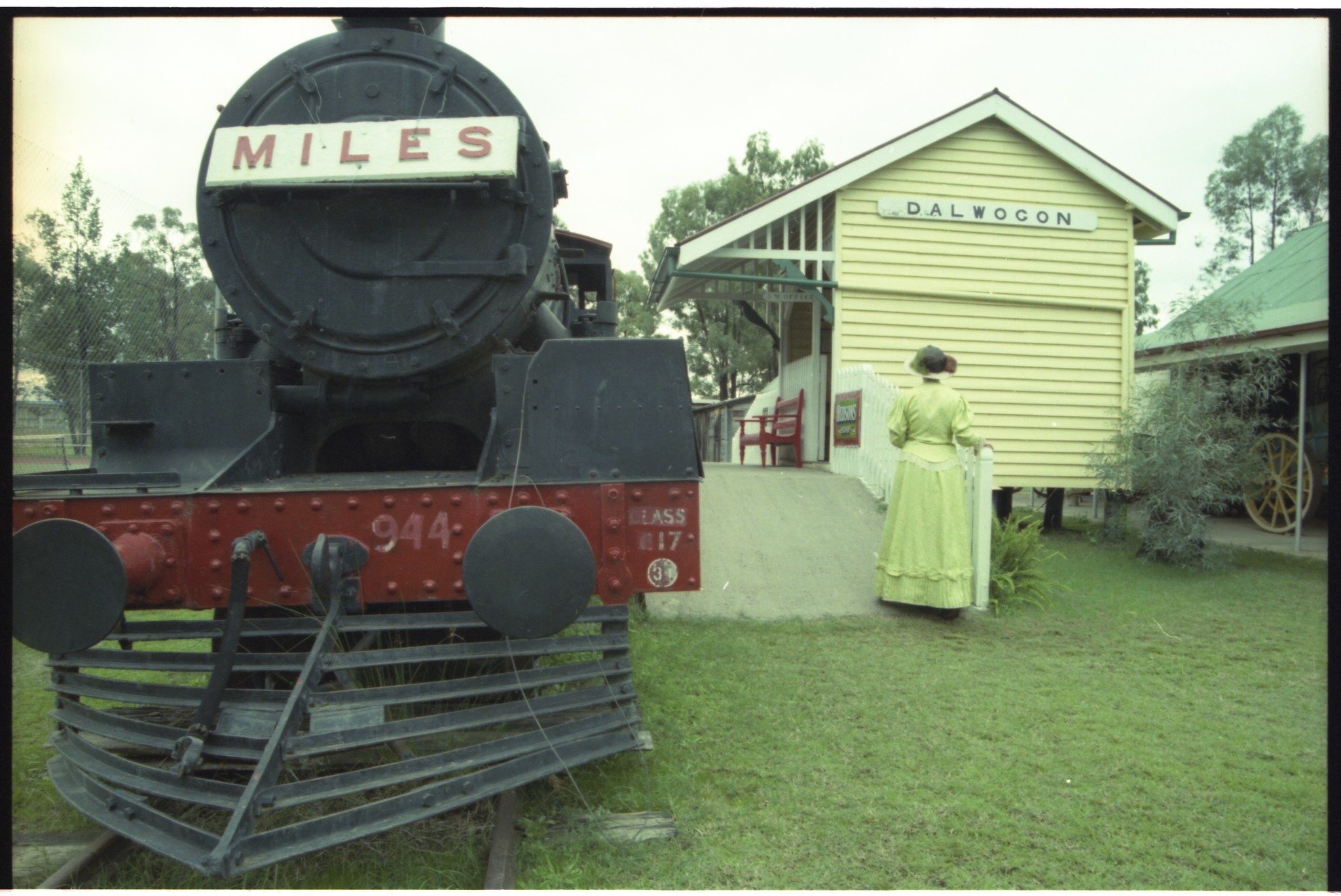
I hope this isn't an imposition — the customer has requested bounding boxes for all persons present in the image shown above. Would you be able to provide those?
[873,344,993,619]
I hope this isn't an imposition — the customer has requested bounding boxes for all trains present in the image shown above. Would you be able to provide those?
[14,16,702,887]
